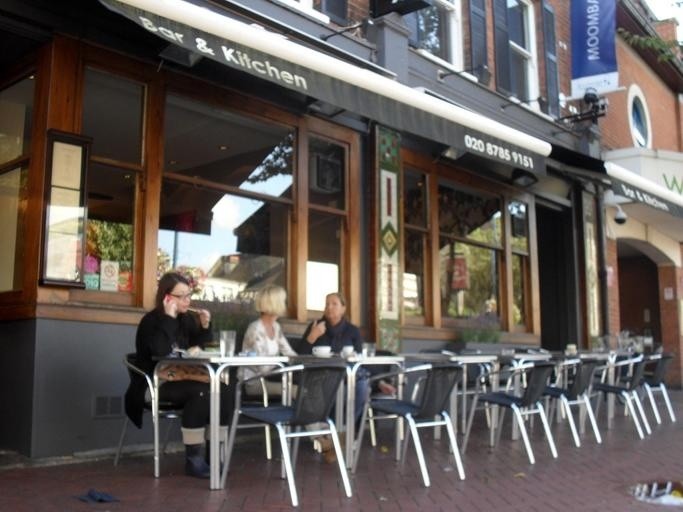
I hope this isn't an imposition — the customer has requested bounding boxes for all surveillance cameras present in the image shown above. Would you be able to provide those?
[583,88,599,104]
[613,211,627,224]
[359,14,377,31]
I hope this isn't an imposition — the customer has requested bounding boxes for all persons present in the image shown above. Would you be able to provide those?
[301,293,397,465]
[240,284,344,462]
[135,273,234,479]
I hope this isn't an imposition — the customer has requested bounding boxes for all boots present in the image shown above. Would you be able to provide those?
[180,426,211,478]
[206,425,230,474]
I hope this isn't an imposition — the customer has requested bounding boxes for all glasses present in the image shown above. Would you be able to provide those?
[171,293,193,301]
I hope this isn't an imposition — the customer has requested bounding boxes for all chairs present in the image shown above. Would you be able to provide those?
[462,361,558,464]
[584,352,651,439]
[530,358,602,446]
[624,353,676,424]
[113,352,228,477]
[418,349,490,427]
[354,363,466,487]
[371,350,406,399]
[220,362,352,506]
[238,366,297,460]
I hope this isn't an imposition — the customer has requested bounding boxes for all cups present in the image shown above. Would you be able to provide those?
[312,345,332,359]
[566,343,578,357]
[361,343,376,357]
[341,345,354,359]
[219,329,238,360]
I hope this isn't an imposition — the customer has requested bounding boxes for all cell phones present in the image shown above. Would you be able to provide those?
[164,295,171,302]
